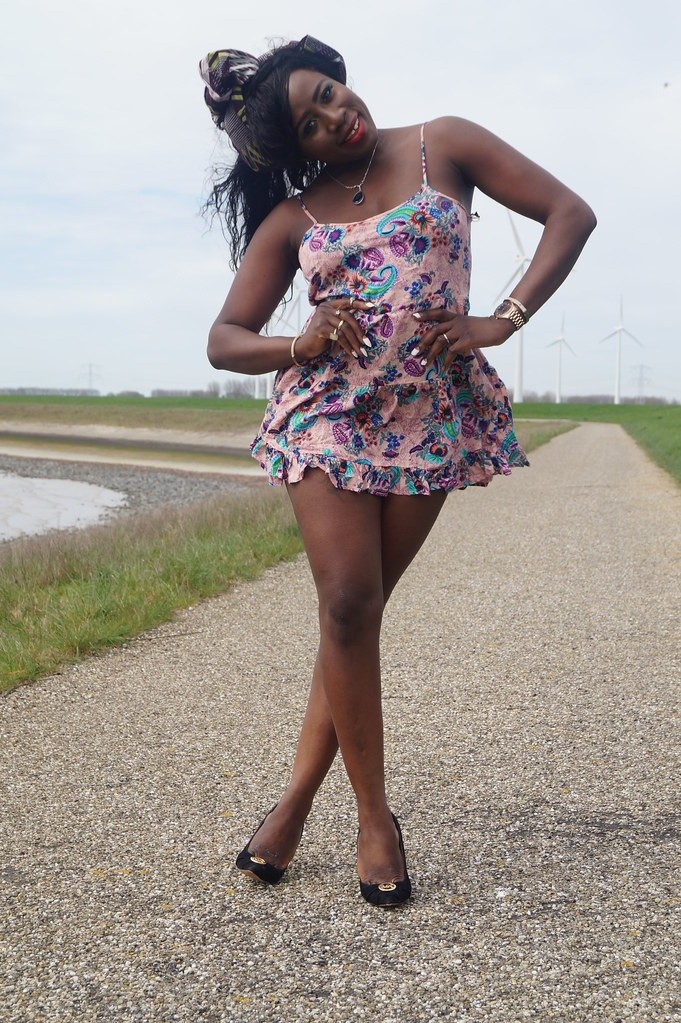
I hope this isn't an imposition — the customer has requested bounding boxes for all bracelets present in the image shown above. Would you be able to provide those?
[503,297,529,323]
[290,334,303,367]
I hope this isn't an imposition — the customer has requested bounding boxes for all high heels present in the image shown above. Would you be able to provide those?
[236,802,306,885]
[356,813,410,909]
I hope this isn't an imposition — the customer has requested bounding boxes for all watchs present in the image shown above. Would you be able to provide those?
[494,299,524,331]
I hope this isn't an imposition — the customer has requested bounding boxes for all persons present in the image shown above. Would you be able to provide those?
[196,32,599,908]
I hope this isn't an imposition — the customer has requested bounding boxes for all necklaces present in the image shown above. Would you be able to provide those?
[324,129,380,206]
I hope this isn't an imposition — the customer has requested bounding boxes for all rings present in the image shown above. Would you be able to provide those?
[335,308,341,318]
[337,319,346,330]
[349,299,355,308]
[329,328,338,340]
[442,333,450,344]
